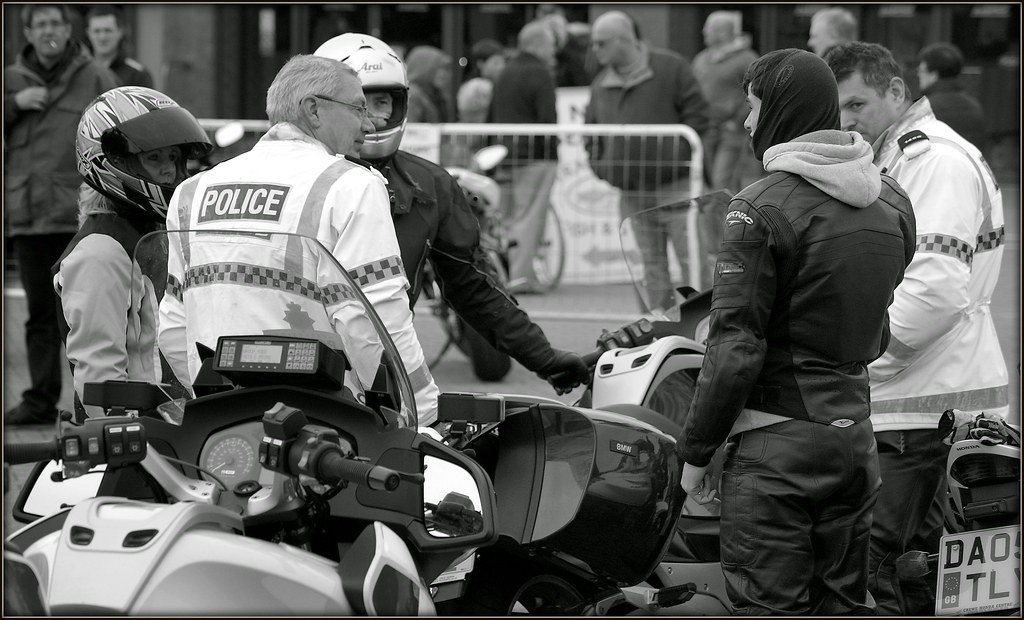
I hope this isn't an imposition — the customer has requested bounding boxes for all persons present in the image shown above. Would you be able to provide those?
[681,49,916,617]
[917,43,993,166]
[822,43,1009,618]
[484,21,558,294]
[807,8,858,58]
[50,87,214,426]
[581,11,715,309]
[4,4,123,426]
[85,7,155,89]
[537,6,589,86]
[464,36,505,80]
[156,55,451,438]
[404,44,455,122]
[691,11,760,192]
[311,32,585,395]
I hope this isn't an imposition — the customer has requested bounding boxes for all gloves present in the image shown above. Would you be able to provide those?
[536,347,592,395]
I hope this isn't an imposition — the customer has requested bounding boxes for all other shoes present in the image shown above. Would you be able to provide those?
[5,403,58,426]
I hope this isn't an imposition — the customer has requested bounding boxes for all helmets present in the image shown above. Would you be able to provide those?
[72,84,214,227]
[315,31,410,158]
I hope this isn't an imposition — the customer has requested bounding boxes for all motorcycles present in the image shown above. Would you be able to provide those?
[894,437,1020,617]
[4,229,496,616]
[551,188,734,616]
[399,87,514,384]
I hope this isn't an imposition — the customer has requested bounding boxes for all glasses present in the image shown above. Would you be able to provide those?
[300,93,369,117]
